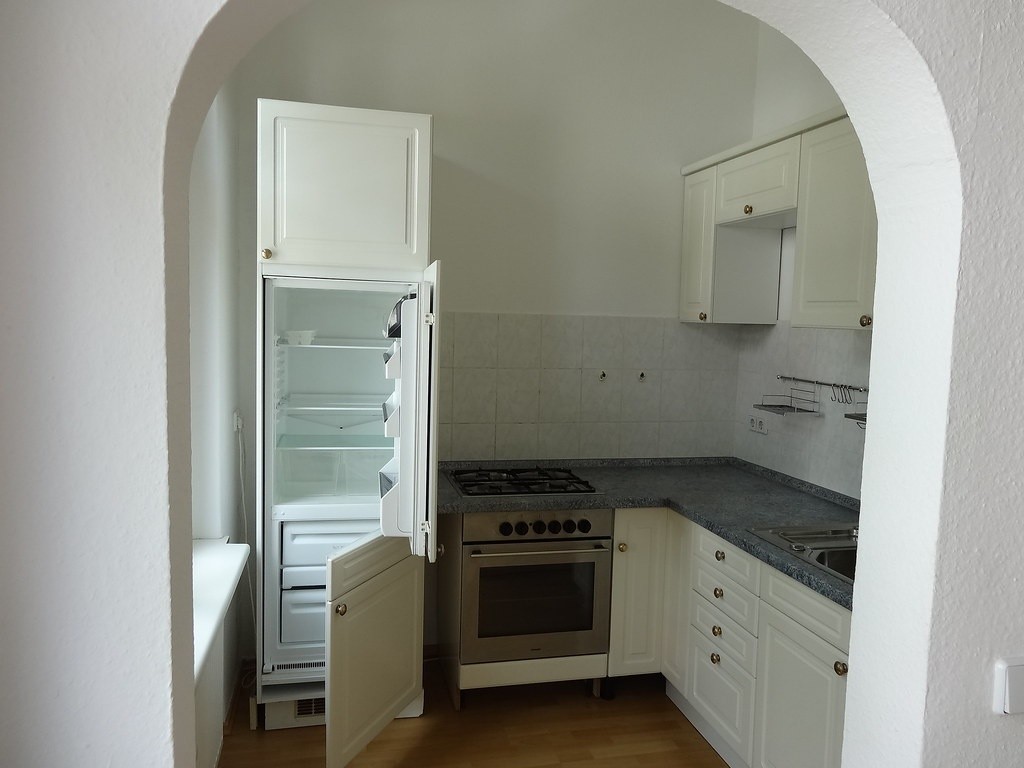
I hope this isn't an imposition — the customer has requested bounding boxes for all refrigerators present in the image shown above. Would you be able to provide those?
[260,259,442,768]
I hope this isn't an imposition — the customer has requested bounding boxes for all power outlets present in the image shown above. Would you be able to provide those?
[748,415,768,435]
[234,409,241,431]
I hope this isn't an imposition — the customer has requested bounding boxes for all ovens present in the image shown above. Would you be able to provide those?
[458,539,614,665]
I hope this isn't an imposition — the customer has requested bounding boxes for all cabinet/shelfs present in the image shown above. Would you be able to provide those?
[684,525,763,768]
[715,136,800,228]
[608,507,668,678]
[258,98,434,276]
[671,167,716,323]
[790,118,878,330]
[661,507,698,695]
[757,562,851,768]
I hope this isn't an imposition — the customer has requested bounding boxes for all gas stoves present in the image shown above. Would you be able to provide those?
[441,467,613,541]
